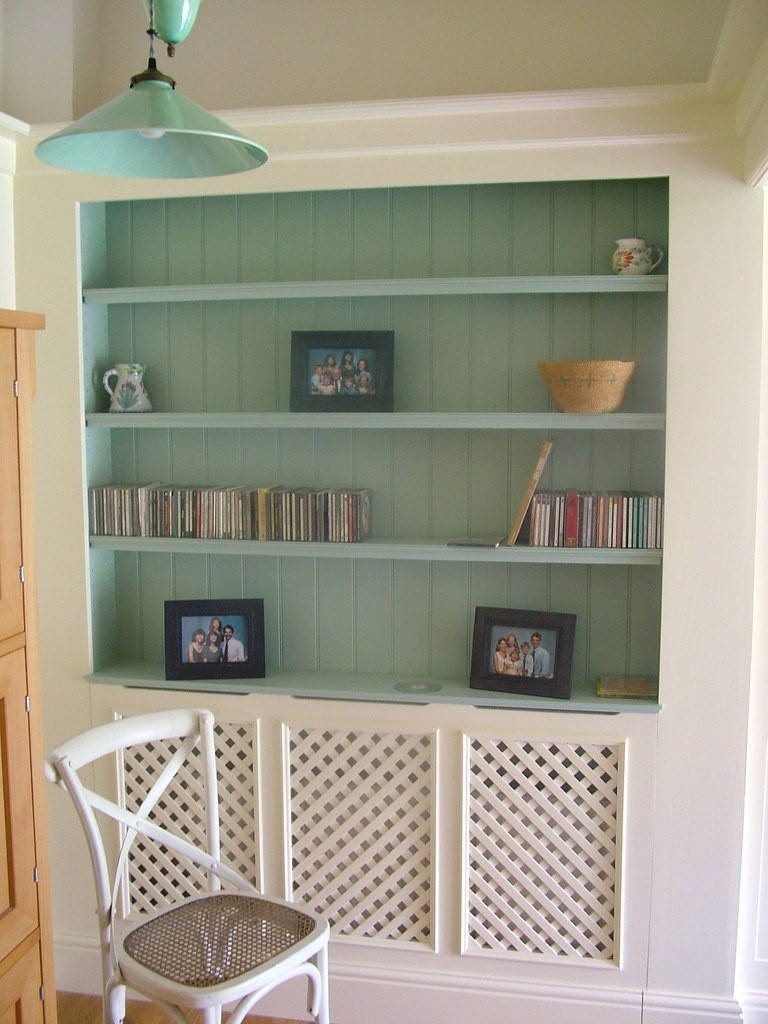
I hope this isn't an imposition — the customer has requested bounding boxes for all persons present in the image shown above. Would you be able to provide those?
[189,619,244,662]
[493,632,550,677]
[311,351,375,395]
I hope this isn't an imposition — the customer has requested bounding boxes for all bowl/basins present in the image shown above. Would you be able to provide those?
[538,358,636,414]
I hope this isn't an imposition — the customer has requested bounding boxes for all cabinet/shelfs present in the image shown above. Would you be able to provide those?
[81,273,671,718]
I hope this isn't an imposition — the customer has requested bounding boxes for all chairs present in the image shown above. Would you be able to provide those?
[43,707,331,1024]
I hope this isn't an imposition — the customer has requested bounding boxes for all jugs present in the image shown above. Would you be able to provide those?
[101,363,155,414]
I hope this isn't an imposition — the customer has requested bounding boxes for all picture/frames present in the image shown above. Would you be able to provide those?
[469,605,576,699]
[290,331,395,412]
[164,599,269,682]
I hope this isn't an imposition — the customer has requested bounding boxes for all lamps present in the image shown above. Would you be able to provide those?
[34,0,269,180]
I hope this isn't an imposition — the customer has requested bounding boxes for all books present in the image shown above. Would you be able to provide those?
[529,489,664,549]
[88,481,372,543]
[596,676,658,699]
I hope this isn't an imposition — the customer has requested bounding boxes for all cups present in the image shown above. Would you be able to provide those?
[613,237,664,275]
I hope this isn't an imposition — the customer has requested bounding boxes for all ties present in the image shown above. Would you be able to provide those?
[223,640,228,663]
[523,656,526,668]
[335,380,338,395]
[532,651,535,677]
